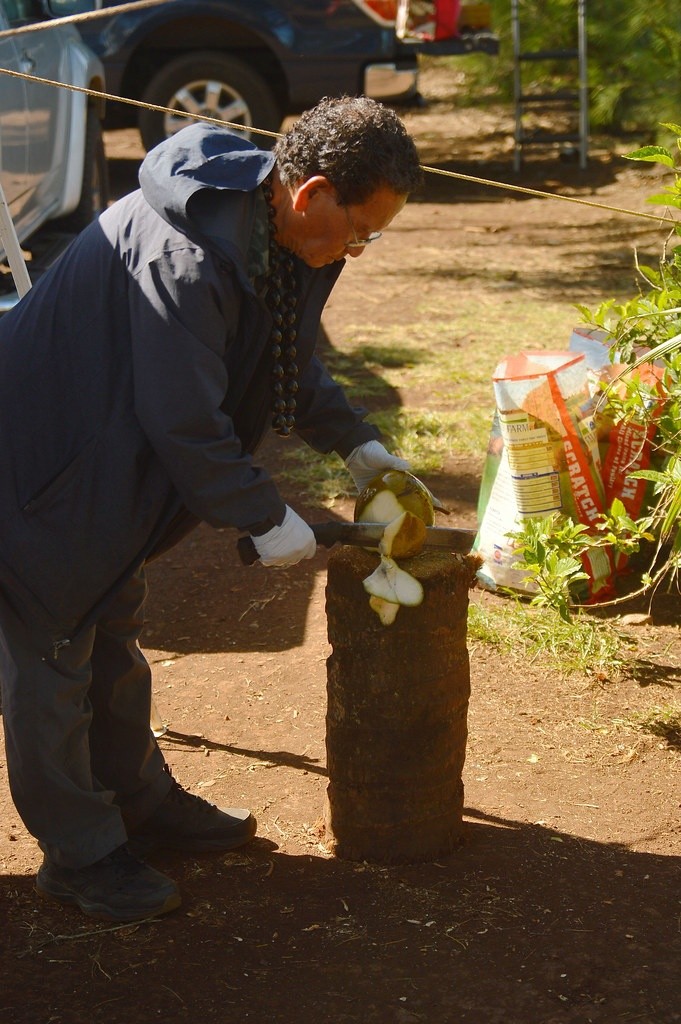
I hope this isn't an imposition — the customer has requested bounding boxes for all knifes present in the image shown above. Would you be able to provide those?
[237,521,477,570]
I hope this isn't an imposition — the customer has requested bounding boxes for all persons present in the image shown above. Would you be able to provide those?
[0,93,447,922]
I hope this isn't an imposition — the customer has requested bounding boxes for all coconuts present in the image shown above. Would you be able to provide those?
[349,469,435,561]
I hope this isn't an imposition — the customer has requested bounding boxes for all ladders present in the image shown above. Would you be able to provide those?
[511,0,588,187]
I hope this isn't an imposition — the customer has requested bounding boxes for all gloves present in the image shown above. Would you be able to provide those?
[344,440,443,513]
[249,504,317,567]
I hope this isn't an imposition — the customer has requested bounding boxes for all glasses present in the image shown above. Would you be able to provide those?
[335,188,383,247]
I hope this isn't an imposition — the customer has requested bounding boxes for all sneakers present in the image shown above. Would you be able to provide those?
[36,841,182,922]
[126,764,257,851]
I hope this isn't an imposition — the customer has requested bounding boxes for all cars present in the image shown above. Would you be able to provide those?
[0,0,108,262]
[80,0,422,157]
